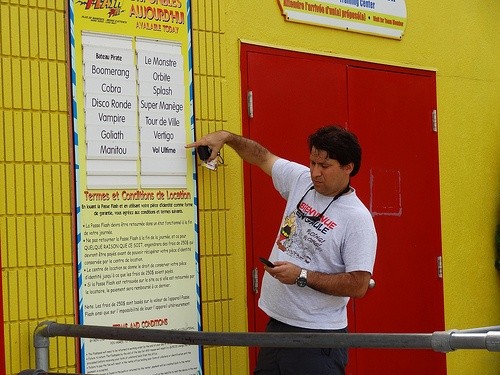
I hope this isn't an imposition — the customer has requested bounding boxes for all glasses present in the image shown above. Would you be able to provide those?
[294,188,339,224]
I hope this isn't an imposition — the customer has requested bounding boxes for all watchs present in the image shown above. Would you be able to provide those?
[296,269,307,287]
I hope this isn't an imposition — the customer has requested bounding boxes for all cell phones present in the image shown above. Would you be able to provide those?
[260,257,275,268]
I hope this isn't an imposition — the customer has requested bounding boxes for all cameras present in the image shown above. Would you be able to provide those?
[197,145,220,160]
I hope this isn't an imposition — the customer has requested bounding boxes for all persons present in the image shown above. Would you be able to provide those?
[185,123,379,375]
